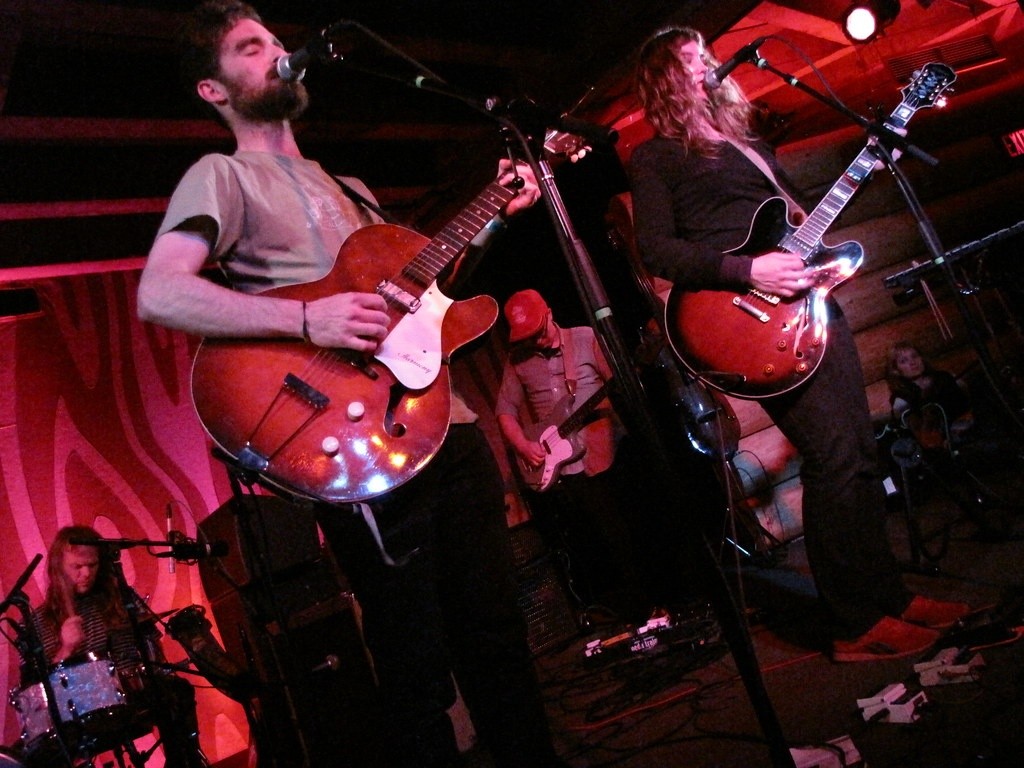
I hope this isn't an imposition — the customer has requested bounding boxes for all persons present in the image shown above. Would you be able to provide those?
[137,2,561,768]
[497,289,670,627]
[16,526,207,768]
[630,26,1024,662]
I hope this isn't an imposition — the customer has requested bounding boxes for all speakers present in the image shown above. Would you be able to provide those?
[197,492,432,768]
[509,520,581,655]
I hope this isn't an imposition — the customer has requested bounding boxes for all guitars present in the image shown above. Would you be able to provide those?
[187,124,595,505]
[662,61,960,401]
[513,313,667,494]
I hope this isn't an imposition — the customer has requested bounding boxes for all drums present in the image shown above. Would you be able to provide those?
[7,650,136,758]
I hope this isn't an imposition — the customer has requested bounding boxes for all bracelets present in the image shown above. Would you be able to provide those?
[302,300,312,342]
[484,219,504,233]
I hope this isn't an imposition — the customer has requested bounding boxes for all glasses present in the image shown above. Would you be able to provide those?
[513,329,547,348]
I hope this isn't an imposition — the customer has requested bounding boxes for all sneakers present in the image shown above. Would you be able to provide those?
[832,615,939,662]
[893,593,970,630]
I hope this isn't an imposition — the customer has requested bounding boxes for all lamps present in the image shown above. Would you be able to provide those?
[842,0,901,43]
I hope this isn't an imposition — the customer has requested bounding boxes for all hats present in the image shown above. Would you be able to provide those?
[502,289,547,341]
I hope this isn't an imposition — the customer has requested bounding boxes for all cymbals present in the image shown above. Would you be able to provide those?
[101,606,180,639]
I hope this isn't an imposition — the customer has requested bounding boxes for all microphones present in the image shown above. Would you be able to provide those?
[7,618,44,654]
[166,503,174,574]
[158,543,230,558]
[276,19,347,82]
[705,37,766,89]
